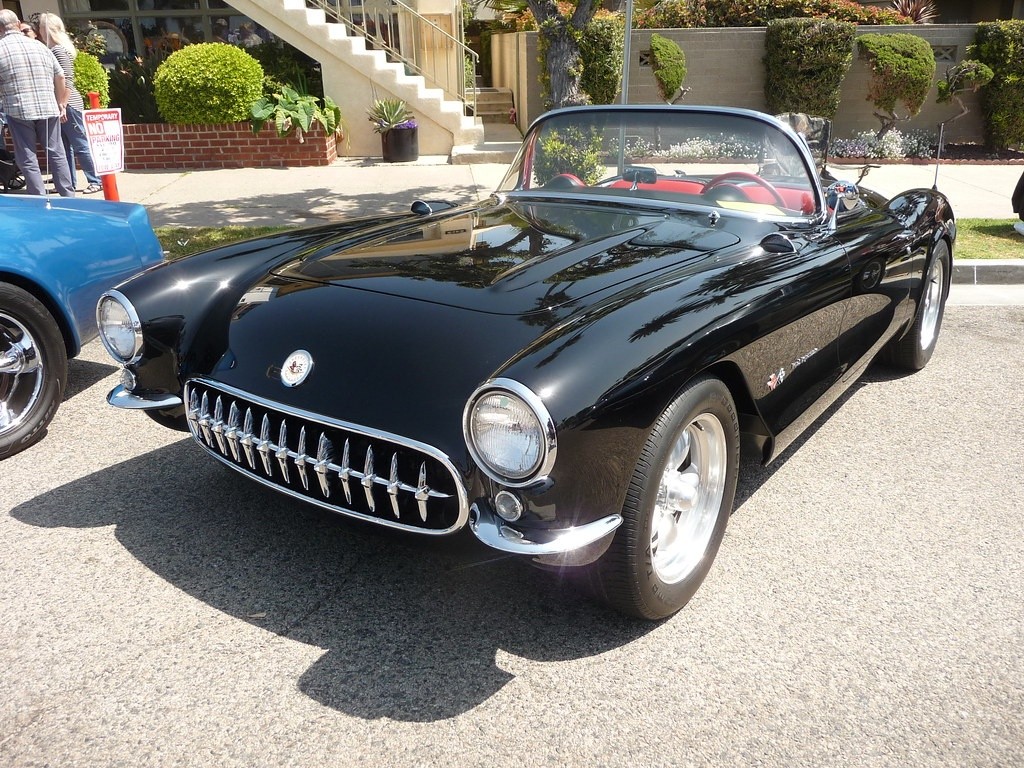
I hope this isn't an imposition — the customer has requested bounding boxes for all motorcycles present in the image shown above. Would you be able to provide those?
[0,146,28,190]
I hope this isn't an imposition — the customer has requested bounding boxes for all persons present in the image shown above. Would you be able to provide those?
[213,19,264,47]
[0,9,103,197]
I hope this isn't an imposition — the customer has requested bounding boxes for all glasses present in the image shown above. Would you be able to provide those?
[20,28,34,34]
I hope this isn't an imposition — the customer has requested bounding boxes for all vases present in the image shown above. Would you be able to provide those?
[382,129,418,162]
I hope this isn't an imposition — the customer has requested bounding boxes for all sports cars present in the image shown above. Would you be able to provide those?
[95,104,956,621]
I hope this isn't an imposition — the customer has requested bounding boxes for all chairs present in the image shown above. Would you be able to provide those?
[739,182,833,214]
[611,179,708,196]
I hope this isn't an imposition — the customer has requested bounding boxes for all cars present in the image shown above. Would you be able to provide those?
[0,192,170,462]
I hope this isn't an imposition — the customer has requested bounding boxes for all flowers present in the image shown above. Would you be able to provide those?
[366,97,416,131]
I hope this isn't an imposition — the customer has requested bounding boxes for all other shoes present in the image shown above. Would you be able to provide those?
[83,184,103,194]
[50,184,60,193]
